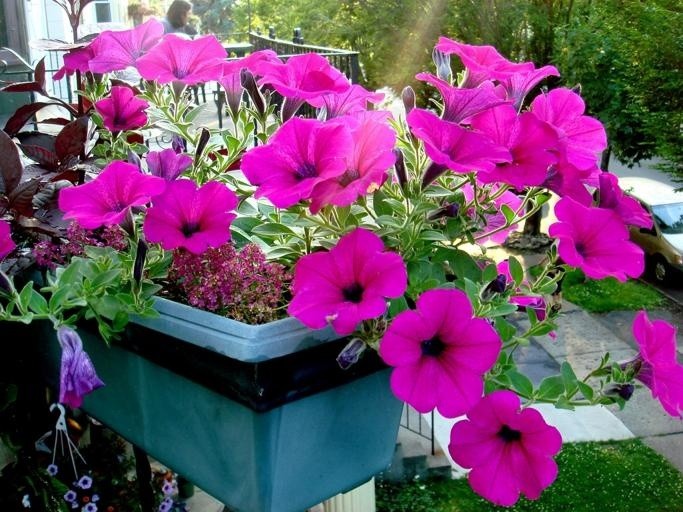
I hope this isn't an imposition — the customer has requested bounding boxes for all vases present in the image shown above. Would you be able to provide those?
[28,267,405,512]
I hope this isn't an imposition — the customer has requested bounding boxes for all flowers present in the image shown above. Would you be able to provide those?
[0,19,683,506]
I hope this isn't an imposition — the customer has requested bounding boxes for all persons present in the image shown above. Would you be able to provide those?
[162,0,194,36]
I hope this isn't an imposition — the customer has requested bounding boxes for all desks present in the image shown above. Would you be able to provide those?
[223,42,254,59]
